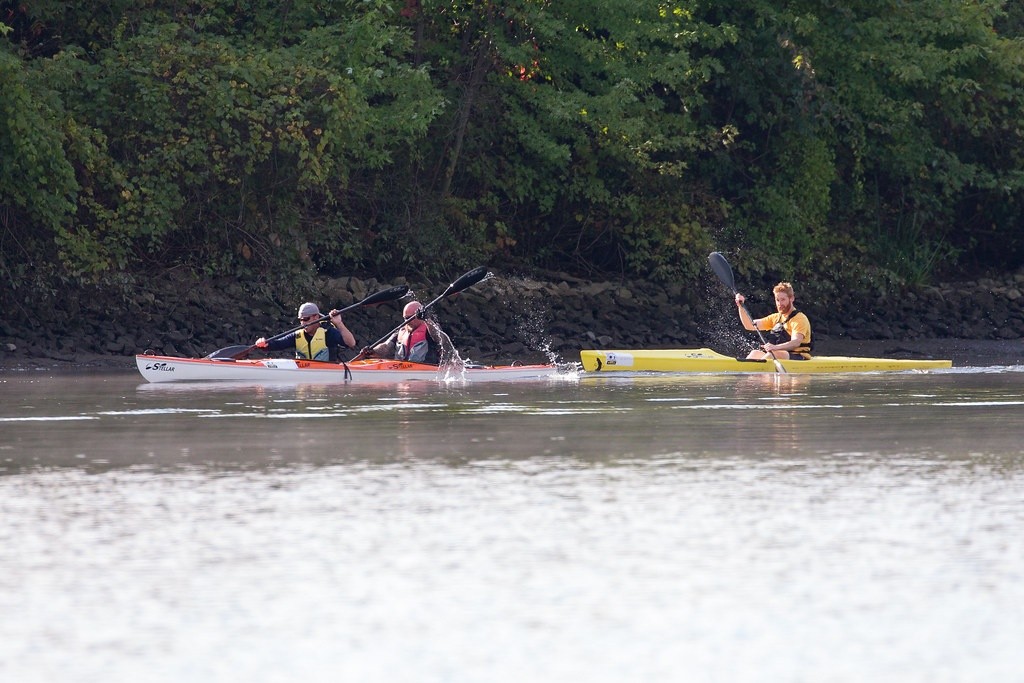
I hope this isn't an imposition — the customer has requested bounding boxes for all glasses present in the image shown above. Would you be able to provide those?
[299,317,311,321]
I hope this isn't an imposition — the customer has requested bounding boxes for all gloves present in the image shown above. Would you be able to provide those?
[360,346,374,356]
[415,305,429,320]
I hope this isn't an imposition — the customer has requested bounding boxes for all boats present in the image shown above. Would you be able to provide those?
[576,345,954,374]
[136,348,561,386]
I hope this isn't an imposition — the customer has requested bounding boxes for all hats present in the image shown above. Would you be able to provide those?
[298,302,325,318]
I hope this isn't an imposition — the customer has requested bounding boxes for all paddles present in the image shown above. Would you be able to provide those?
[345,264,492,362]
[708,251,786,373]
[210,283,410,358]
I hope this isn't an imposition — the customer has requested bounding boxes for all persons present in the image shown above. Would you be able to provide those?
[735,282,811,360]
[360,301,441,364]
[255,302,356,362]
